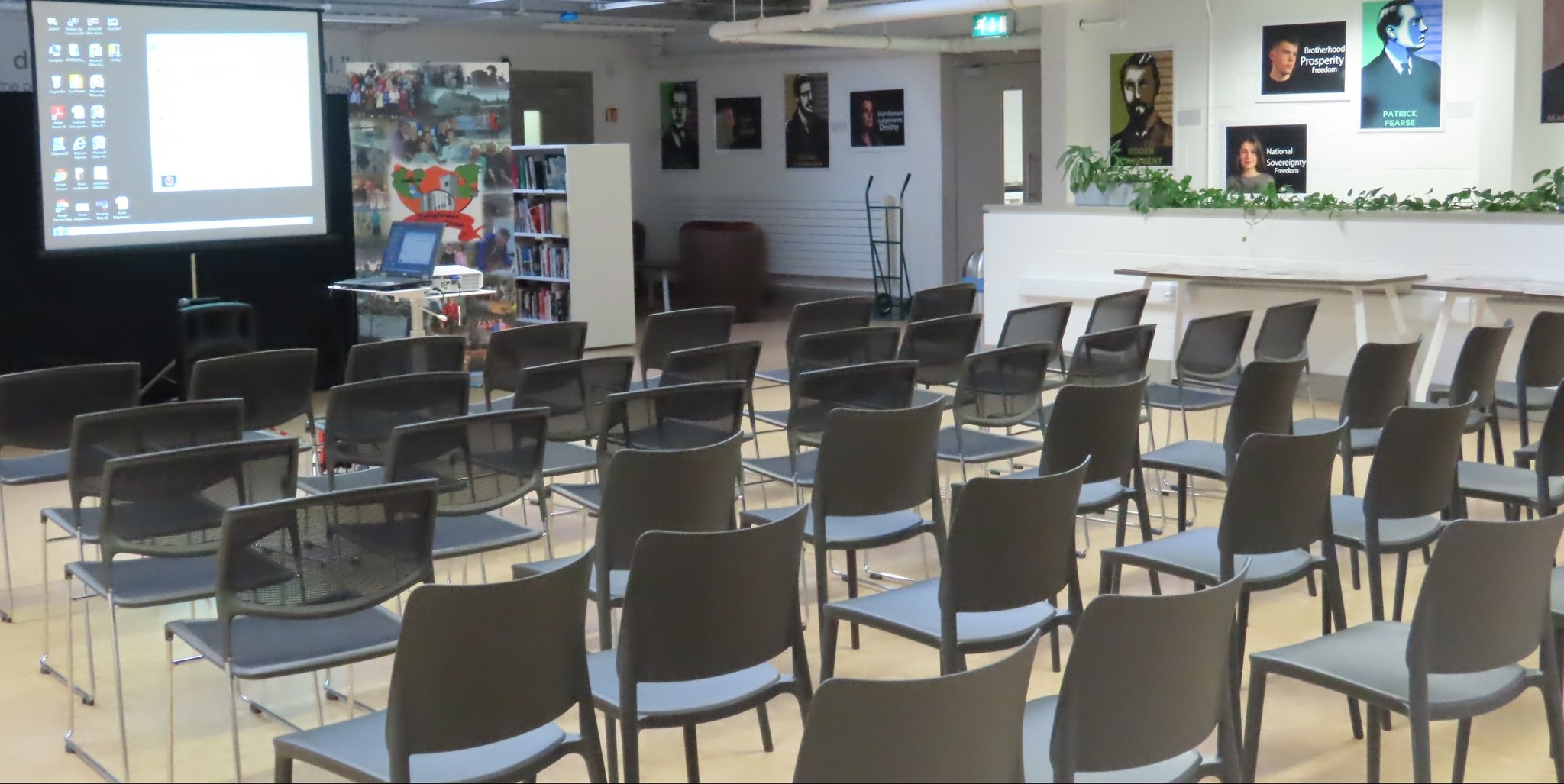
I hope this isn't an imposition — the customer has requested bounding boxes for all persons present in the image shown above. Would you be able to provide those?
[1261,31,1304,93]
[1225,134,1276,195]
[343,59,516,322]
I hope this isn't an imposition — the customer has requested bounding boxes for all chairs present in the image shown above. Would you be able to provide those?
[0,302,1564,784]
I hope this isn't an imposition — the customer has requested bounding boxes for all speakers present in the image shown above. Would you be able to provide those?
[176,302,256,401]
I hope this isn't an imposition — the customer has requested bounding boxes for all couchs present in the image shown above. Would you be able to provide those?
[674,220,769,323]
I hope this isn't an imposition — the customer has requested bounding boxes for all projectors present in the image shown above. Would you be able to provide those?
[431,265,483,294]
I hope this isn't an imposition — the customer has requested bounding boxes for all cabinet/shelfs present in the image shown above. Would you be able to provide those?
[478,143,637,350]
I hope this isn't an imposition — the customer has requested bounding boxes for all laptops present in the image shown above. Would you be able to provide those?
[334,221,447,292]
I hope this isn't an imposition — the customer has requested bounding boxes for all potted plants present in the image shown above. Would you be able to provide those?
[1055,139,1171,206]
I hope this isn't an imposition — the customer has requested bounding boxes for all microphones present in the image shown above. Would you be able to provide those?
[178,295,220,309]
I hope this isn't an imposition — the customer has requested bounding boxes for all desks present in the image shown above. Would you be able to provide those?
[633,259,678,313]
[1114,261,1428,385]
[327,284,497,338]
[1411,277,1564,402]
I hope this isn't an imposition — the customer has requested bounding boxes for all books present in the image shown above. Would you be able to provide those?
[511,152,573,328]
